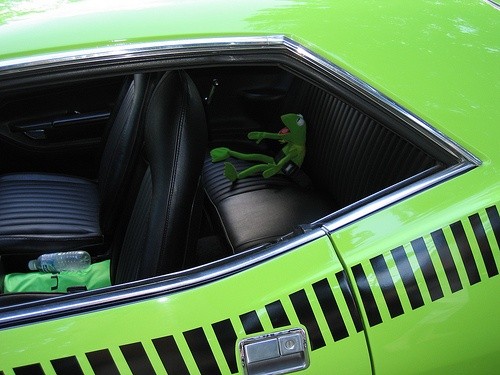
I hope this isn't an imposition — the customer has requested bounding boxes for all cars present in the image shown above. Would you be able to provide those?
[0,0,500,375]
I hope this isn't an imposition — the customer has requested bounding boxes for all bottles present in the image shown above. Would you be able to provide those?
[28,251,91,272]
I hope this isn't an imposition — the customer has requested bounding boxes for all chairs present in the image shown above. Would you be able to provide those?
[0,70,209,307]
[0,73,163,273]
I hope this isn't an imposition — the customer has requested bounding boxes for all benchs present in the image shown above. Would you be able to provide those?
[200,79,435,256]
